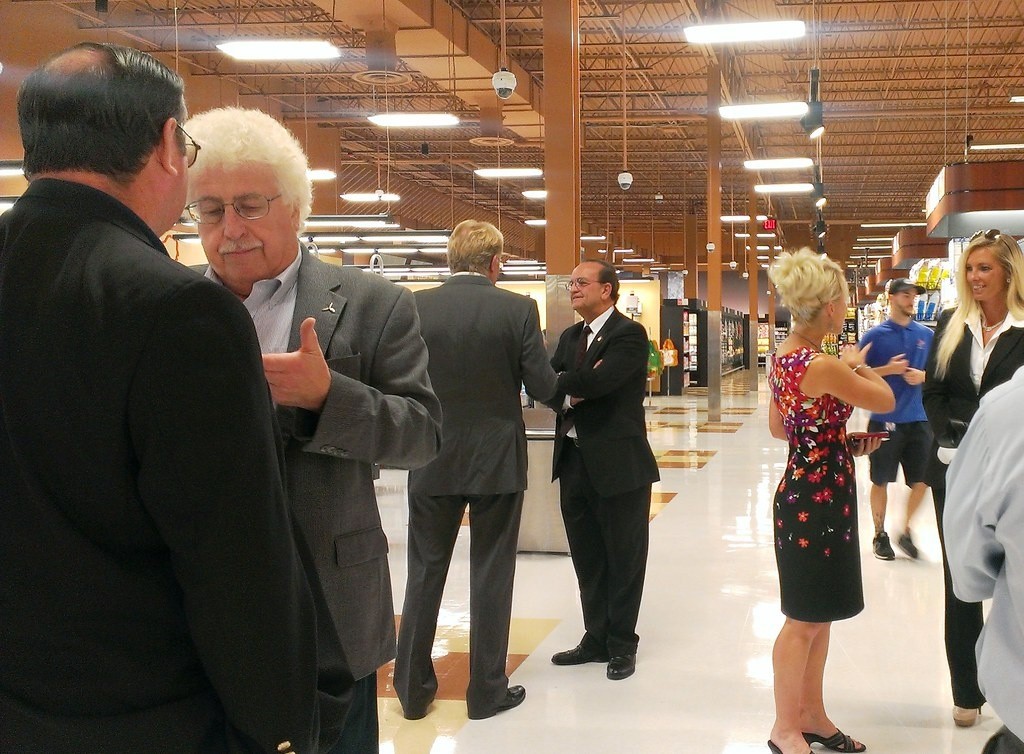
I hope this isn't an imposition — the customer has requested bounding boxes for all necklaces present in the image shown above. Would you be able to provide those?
[792,331,818,348]
[982,319,1004,331]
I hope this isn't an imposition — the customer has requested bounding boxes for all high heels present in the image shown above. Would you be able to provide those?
[953,705,981,727]
[768,740,816,754]
[802,728,866,754]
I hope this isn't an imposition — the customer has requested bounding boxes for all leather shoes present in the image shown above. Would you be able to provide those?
[468,684,526,720]
[550,644,609,665]
[404,697,434,720]
[607,654,636,680]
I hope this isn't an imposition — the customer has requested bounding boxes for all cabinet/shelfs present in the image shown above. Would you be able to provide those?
[660,304,690,397]
[688,310,708,387]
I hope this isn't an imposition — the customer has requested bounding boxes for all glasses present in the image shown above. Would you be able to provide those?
[184,194,281,224]
[968,229,1014,259]
[566,278,604,290]
[178,123,201,168]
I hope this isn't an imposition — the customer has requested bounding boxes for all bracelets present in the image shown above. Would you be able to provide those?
[557,371,565,376]
[852,365,871,371]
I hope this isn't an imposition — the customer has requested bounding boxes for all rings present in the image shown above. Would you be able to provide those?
[864,451,872,455]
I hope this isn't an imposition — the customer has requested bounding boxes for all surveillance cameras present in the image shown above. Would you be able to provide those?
[491,71,517,98]
[655,195,663,204]
[767,291,772,296]
[729,261,737,270]
[705,243,716,252]
[682,269,689,275]
[617,172,633,190]
[742,272,749,279]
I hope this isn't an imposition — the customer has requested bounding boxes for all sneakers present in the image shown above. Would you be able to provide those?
[873,532,896,560]
[898,533,917,558]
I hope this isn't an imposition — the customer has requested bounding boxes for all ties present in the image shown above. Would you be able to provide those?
[555,326,592,439]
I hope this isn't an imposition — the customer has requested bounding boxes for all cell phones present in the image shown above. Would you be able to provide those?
[849,432,891,441]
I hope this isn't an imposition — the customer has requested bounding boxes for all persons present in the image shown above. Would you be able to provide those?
[858,277,936,560]
[393,219,559,720]
[768,248,895,754]
[551,259,660,680]
[922,228,1024,754]
[180,106,443,754]
[0,42,321,754]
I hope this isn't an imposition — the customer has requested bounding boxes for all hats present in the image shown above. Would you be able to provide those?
[888,278,926,295]
